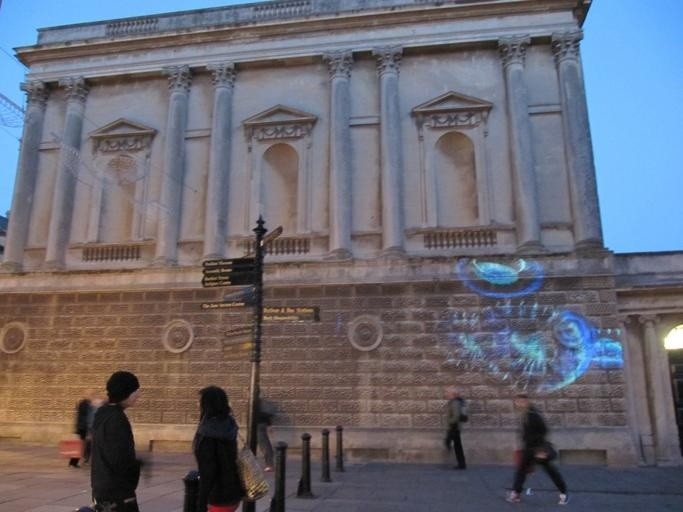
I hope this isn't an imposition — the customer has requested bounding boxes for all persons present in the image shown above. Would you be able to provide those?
[505,392,571,506]
[192,385,242,512]
[248,384,274,472]
[443,385,466,470]
[69,400,91,468]
[90,371,141,512]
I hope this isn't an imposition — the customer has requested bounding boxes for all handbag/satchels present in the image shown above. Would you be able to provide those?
[461,405,468,423]
[235,448,269,502]
[533,442,557,462]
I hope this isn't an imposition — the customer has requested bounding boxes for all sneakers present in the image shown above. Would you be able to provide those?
[506,491,520,503]
[559,492,571,504]
[70,463,80,468]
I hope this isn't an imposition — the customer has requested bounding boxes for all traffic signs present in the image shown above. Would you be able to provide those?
[223,287,255,299]
[262,313,320,322]
[260,226,284,247]
[200,299,256,308]
[220,328,256,362]
[202,257,257,287]
[262,305,319,314]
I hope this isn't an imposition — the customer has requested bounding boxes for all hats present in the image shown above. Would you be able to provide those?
[106,371,139,403]
